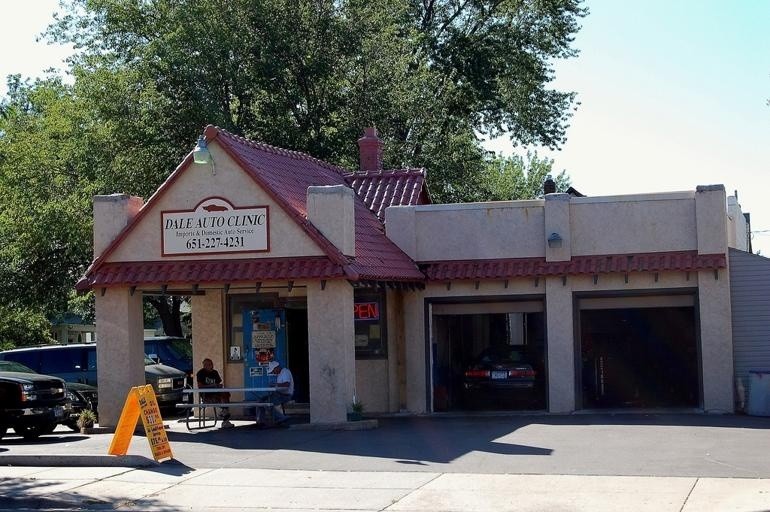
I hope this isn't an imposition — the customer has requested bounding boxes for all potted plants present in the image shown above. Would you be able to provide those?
[78,408,97,428]
[348,400,367,422]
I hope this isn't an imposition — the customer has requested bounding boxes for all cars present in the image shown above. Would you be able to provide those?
[460,344,545,407]
[0,360,98,432]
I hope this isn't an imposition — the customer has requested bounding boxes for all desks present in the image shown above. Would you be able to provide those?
[181,387,282,432]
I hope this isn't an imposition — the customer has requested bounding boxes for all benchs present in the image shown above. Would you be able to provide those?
[173,401,275,428]
[199,397,296,425]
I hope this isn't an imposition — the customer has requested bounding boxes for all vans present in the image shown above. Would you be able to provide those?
[1,337,193,415]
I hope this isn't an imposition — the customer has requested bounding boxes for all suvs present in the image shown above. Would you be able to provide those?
[1,372,72,439]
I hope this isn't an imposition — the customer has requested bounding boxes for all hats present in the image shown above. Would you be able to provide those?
[267,361,279,373]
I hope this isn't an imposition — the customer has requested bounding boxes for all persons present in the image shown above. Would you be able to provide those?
[252,361,294,428]
[196,359,234,428]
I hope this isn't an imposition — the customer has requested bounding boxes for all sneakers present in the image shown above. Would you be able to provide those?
[218,411,231,417]
[222,422,234,428]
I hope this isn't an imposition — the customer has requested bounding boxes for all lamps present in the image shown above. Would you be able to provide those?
[193,135,217,176]
[547,233,561,249]
